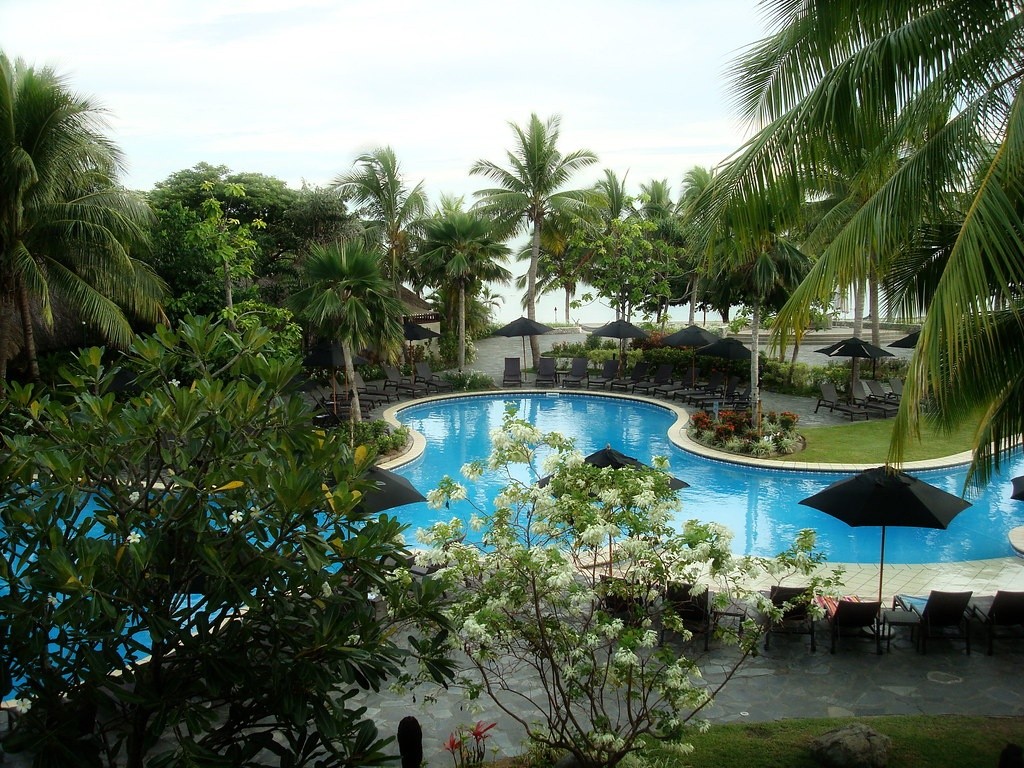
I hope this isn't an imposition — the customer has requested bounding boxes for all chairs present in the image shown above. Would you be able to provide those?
[595,574,665,637]
[328,378,382,409]
[845,381,899,420]
[892,590,972,656]
[610,361,762,413]
[309,391,370,425]
[586,360,620,390]
[817,595,882,655]
[503,357,522,388]
[382,365,429,398]
[535,357,556,388]
[414,361,454,394]
[814,383,869,422]
[962,591,1024,657]
[316,384,373,413]
[562,357,589,388]
[889,379,934,413]
[865,381,906,405]
[660,580,715,653]
[344,372,400,404]
[756,585,816,652]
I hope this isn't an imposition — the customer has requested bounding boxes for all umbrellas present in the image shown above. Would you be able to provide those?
[799,465,974,655]
[536,445,692,578]
[305,461,428,583]
[815,337,896,405]
[889,328,925,350]
[693,337,767,406]
[399,321,441,383]
[1011,473,1024,500]
[592,320,650,371]
[658,324,724,390]
[300,340,369,415]
[491,314,558,381]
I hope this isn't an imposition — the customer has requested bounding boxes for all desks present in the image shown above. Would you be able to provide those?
[881,610,920,653]
[712,597,749,639]
[555,370,570,384]
